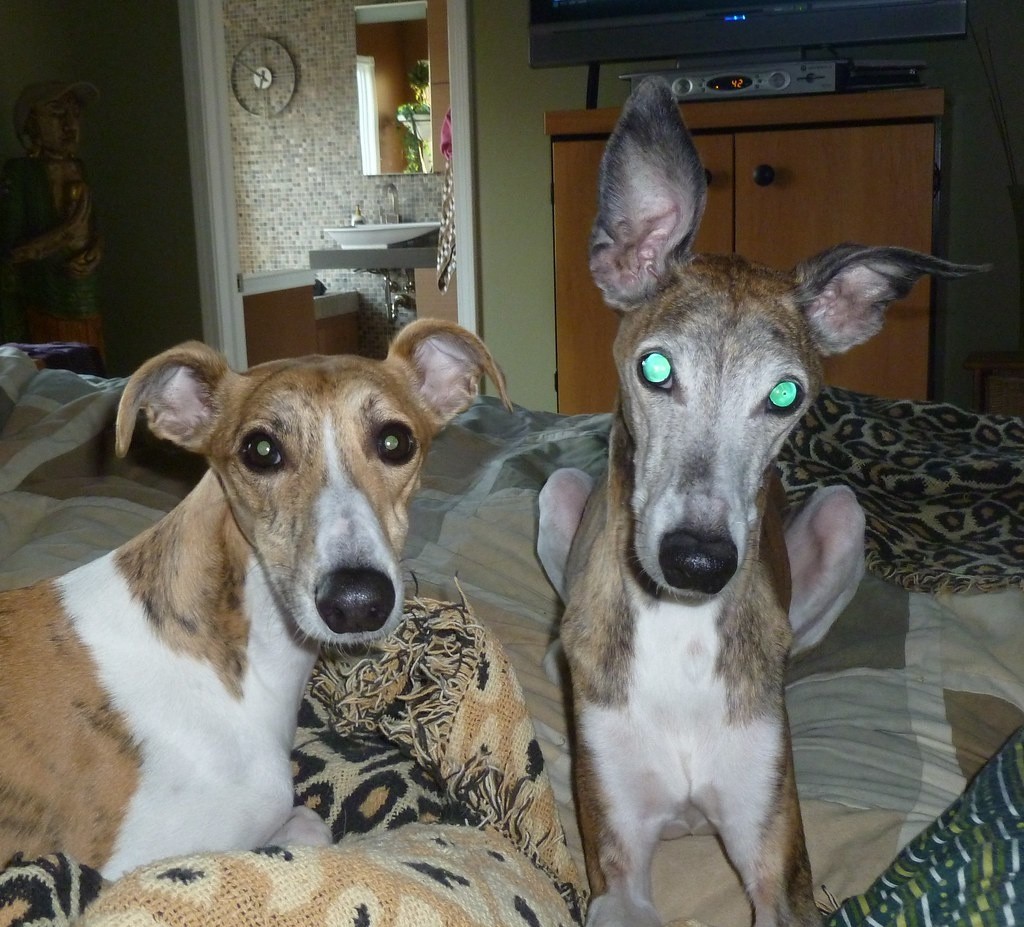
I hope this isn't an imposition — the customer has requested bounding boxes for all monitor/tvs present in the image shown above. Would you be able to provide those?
[528,0,964,68]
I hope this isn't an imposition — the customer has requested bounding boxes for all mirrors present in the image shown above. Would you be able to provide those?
[349,0,451,179]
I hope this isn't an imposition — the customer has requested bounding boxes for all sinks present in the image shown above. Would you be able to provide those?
[323,221,441,250]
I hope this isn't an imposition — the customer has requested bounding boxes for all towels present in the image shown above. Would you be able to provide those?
[441,109,452,160]
[437,161,456,295]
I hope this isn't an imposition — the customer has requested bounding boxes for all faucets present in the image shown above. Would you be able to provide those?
[378,184,401,223]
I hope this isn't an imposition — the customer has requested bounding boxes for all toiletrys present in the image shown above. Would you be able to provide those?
[349,204,366,227]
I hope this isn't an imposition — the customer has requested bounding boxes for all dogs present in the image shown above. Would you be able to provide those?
[0,317,515,882]
[534,73,991,926]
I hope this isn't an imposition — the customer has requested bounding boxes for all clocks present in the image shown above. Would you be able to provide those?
[231,37,297,119]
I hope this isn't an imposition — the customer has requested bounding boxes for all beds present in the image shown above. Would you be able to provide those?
[0,338,1024,927]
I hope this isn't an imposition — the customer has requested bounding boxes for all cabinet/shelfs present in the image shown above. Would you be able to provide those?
[542,86,956,409]
